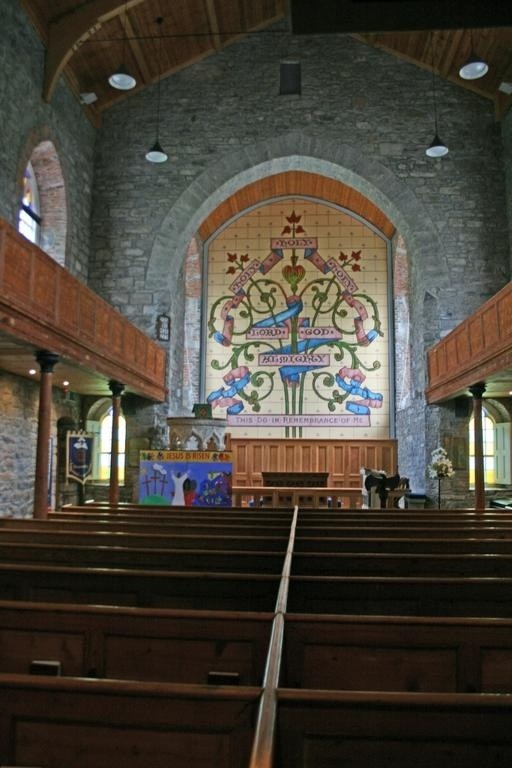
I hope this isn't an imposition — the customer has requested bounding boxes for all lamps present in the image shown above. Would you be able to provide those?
[107,0,137,92]
[145,14,168,163]
[425,28,449,159]
[458,29,489,81]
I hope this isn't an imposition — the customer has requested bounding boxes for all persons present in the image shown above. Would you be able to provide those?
[169,467,192,506]
